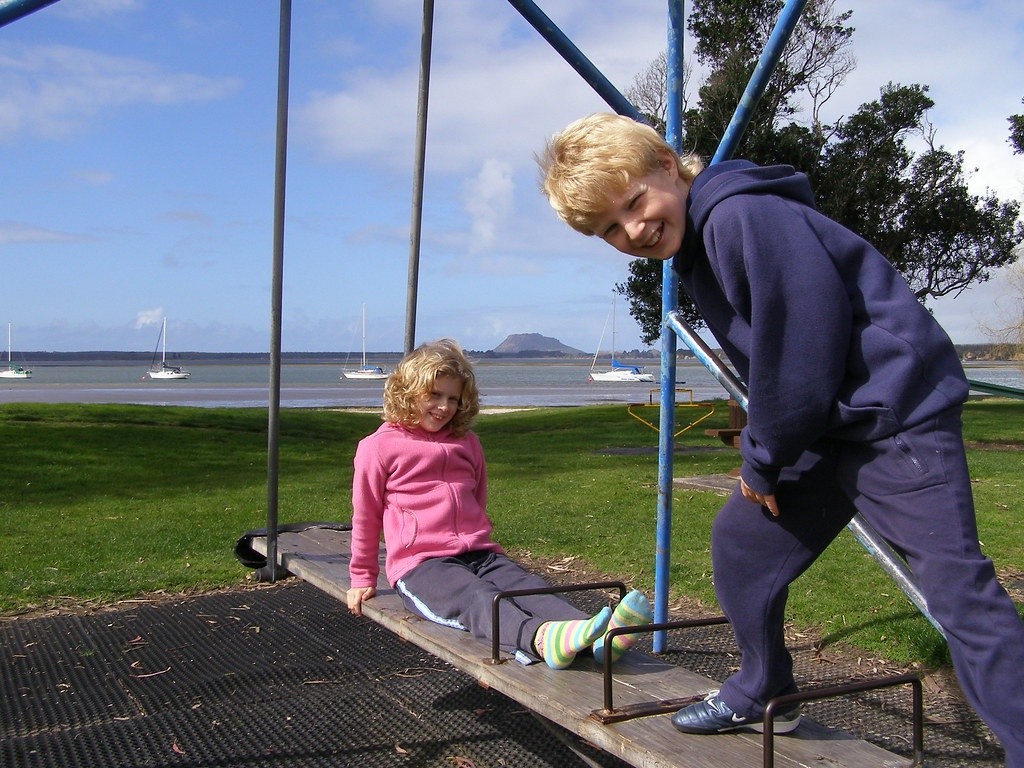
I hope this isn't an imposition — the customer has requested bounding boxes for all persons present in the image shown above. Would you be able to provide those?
[348,339,654,670]
[531,111,1024,768]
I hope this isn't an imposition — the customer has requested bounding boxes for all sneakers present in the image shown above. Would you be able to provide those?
[671,690,801,734]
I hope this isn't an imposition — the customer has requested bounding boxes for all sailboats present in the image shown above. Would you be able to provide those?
[0,320,33,378]
[147,316,191,379]
[586,283,655,382]
[342,303,388,379]
